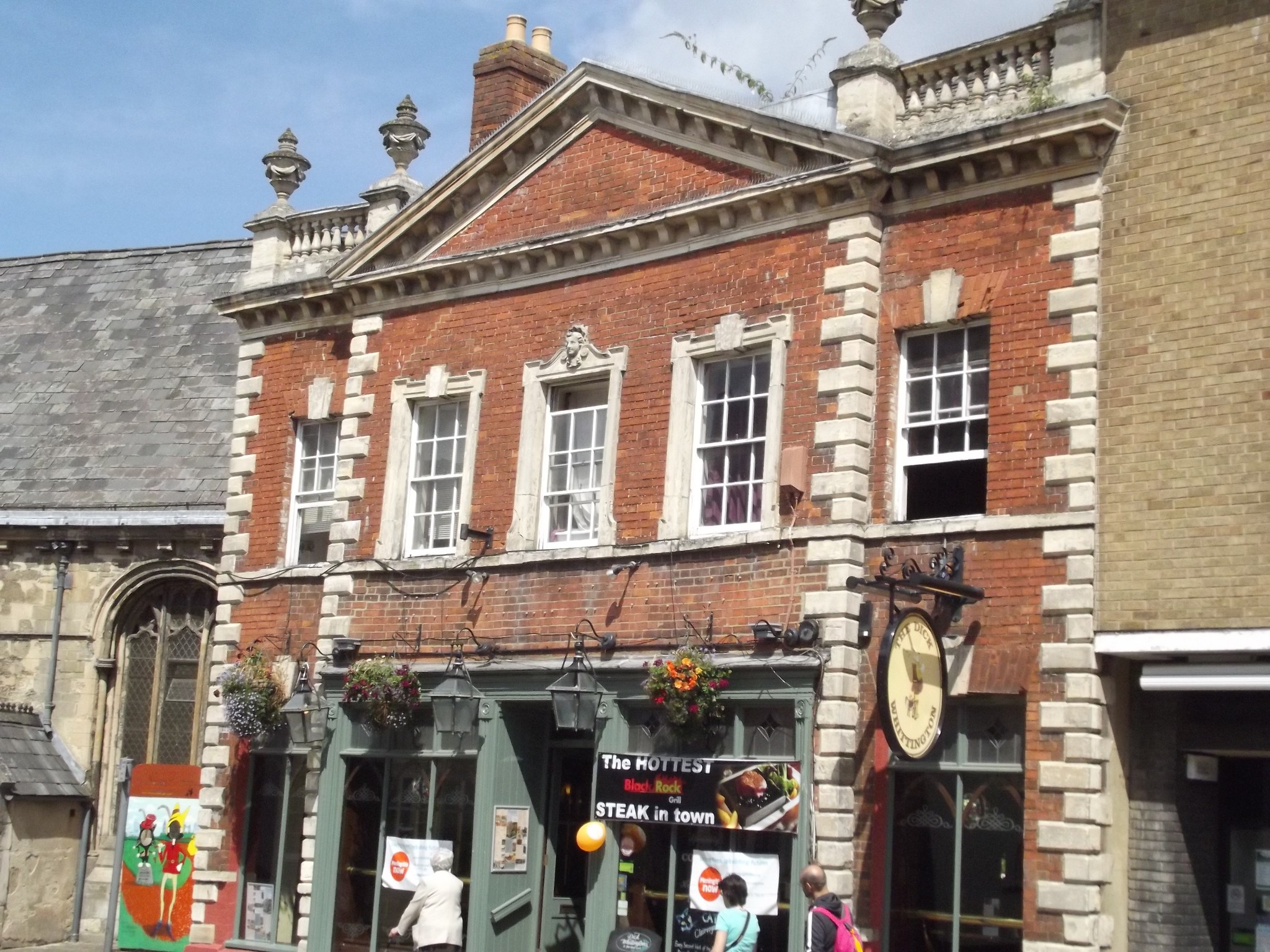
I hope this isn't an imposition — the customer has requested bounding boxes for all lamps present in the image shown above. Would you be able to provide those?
[545,634,610,736]
[427,652,482,737]
[281,668,331,745]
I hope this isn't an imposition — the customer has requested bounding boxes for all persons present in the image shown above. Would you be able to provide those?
[795,863,855,952]
[386,848,466,952]
[560,324,589,369]
[707,872,762,952]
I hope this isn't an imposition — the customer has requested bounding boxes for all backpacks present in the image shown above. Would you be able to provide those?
[809,901,865,952]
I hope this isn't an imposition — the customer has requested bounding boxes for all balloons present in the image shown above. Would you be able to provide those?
[576,821,607,852]
[620,822,647,854]
